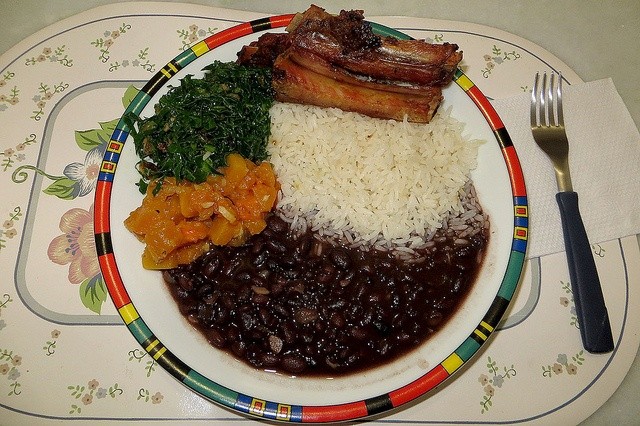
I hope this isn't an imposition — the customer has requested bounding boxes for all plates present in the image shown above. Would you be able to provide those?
[94,14,527,424]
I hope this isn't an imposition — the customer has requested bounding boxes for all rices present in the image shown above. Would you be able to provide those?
[255,76,490,264]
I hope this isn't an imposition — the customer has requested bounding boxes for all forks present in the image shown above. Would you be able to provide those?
[530,71,614,353]
